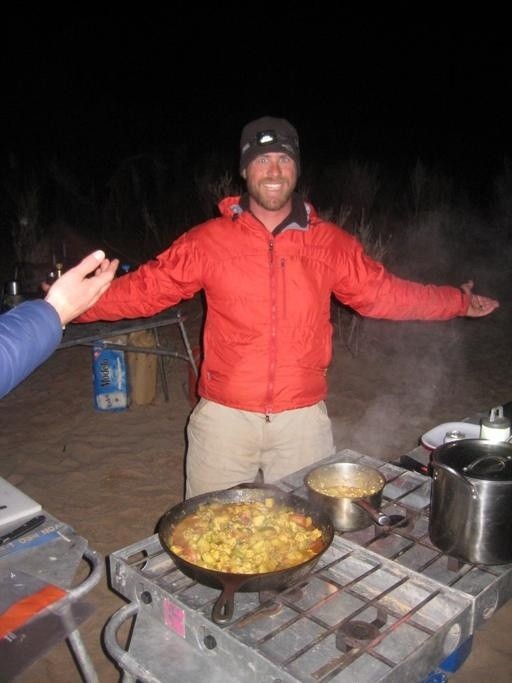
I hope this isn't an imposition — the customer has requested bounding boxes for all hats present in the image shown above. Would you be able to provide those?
[239,117,301,177]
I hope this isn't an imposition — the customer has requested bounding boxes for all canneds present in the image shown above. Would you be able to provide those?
[444,430,465,443]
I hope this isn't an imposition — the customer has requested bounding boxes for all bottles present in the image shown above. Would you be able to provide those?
[442,431,466,445]
[478,405,510,442]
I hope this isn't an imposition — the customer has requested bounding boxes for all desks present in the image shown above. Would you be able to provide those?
[56,307,200,402]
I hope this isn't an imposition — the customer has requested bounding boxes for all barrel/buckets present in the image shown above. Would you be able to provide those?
[100,328,158,406]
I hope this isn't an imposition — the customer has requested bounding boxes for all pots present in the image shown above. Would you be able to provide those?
[154,479,336,633]
[303,455,392,535]
[423,437,512,569]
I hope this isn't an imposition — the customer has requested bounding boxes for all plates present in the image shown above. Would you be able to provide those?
[420,421,512,449]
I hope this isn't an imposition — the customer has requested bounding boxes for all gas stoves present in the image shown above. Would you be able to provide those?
[100,448,512,683]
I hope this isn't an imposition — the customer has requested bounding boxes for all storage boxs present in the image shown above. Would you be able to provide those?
[92,333,128,412]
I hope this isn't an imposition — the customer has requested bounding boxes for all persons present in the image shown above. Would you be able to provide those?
[42,114,504,502]
[1,245,122,403]
